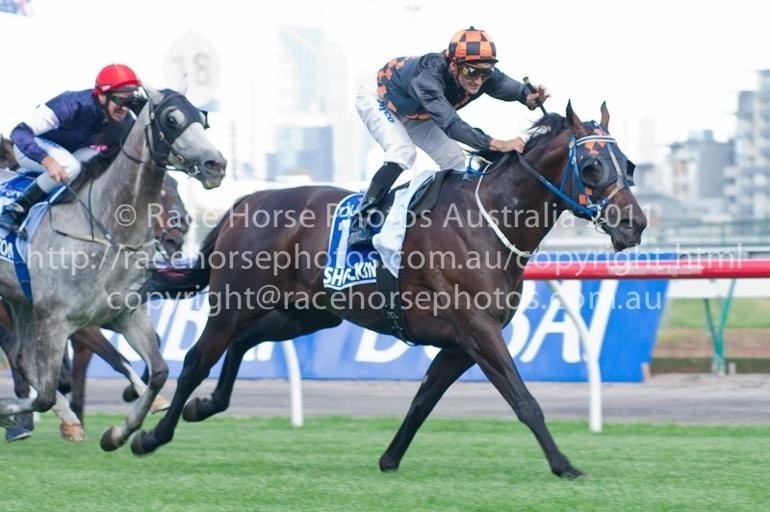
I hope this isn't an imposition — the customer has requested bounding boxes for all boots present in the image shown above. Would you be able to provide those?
[347,179,383,248]
[0,178,48,240]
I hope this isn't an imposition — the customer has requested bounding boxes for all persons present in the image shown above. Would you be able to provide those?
[0,62,143,241]
[348,25,551,245]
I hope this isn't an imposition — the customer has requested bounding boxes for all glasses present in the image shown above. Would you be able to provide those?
[458,63,494,80]
[104,92,134,107]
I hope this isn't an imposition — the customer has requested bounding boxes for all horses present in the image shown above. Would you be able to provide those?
[0,69,228,452]
[130,98,648,483]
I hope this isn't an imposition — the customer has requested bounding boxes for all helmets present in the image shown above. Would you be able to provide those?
[446,27,499,69]
[92,64,142,97]
